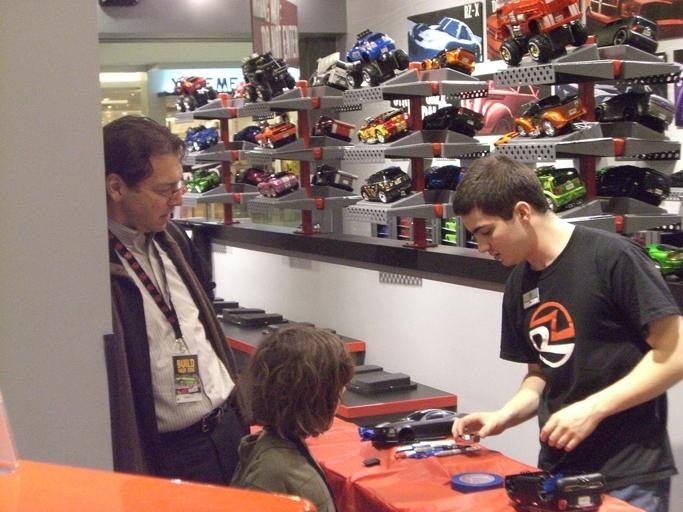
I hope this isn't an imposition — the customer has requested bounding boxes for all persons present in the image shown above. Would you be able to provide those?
[103,114,252,485]
[451,154,683,511]
[229,325,354,512]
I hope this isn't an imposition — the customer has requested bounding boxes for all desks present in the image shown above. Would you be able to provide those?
[248,412,653,512]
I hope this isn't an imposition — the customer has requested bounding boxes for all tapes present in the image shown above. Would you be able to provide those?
[450,471,504,492]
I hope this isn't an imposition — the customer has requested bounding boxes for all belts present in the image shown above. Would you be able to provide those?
[155,393,234,448]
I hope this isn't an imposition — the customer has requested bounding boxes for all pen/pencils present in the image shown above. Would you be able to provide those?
[393,436,481,461]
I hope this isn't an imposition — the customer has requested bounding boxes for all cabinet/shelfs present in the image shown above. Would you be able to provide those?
[164,44,683,236]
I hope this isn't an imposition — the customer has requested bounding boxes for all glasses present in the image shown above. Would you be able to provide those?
[136,181,189,201]
